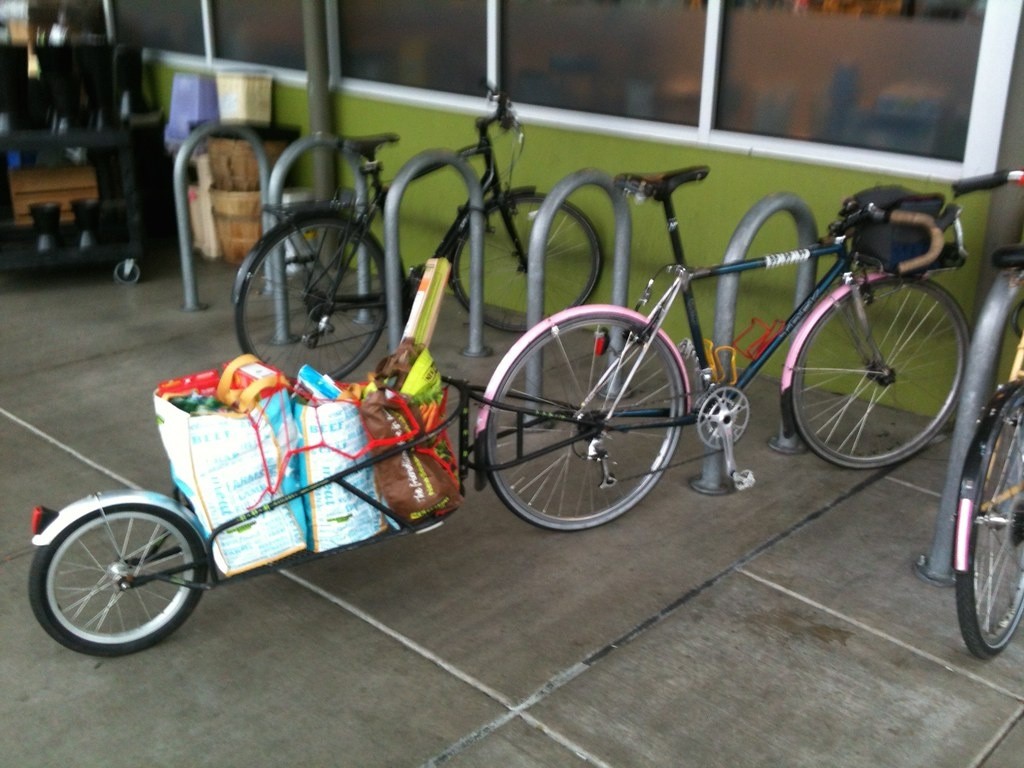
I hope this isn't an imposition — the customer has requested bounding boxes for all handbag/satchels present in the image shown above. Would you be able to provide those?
[359,335,464,523]
[850,184,951,264]
[151,354,308,580]
[259,362,406,554]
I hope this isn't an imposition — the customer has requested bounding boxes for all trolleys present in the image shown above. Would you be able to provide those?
[2,41,146,286]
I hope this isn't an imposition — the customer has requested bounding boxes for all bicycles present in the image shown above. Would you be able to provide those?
[228,83,605,387]
[472,165,975,533]
[949,168,1024,659]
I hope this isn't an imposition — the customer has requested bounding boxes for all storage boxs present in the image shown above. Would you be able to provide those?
[9,164,98,225]
[215,71,273,122]
[166,71,216,144]
